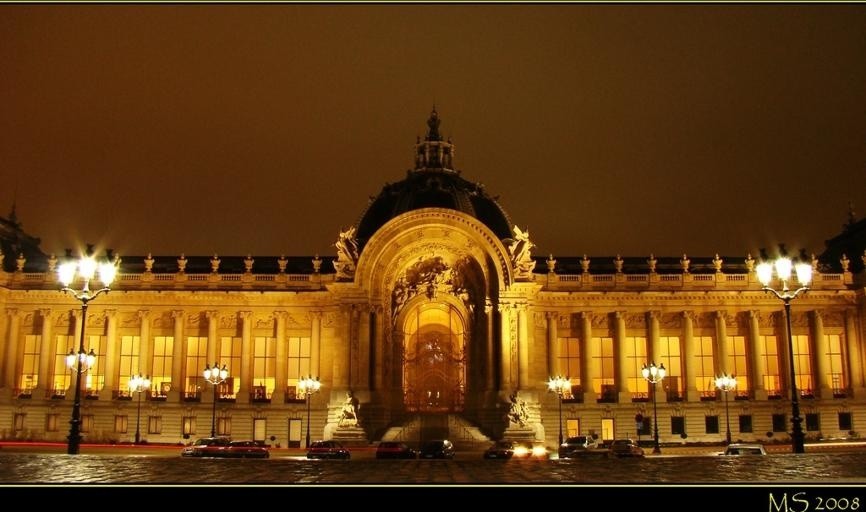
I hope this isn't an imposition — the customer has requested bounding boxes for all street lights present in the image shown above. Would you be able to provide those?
[52,251,123,454]
[639,359,669,454]
[752,251,818,452]
[713,371,740,444]
[296,373,321,448]
[202,359,230,436]
[63,346,95,374]
[126,370,150,445]
[548,375,574,445]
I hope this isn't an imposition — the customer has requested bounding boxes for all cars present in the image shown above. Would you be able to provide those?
[418,439,457,460]
[375,440,417,458]
[480,440,523,459]
[558,434,597,459]
[608,438,646,455]
[306,440,353,458]
[193,437,230,457]
[180,440,196,457]
[227,440,271,458]
[716,443,768,457]
